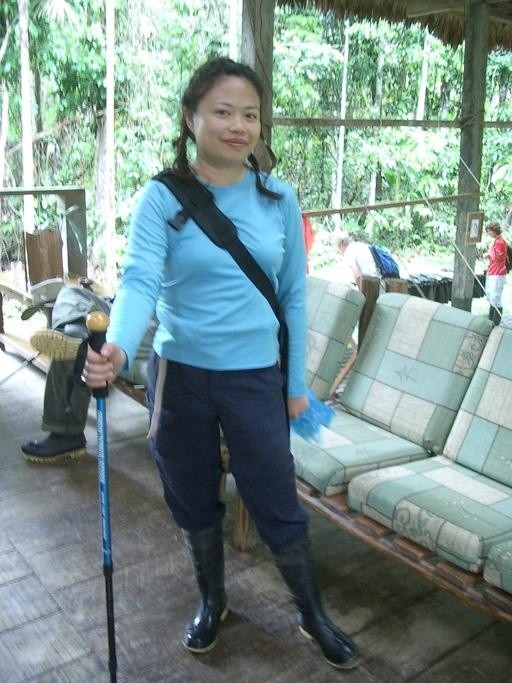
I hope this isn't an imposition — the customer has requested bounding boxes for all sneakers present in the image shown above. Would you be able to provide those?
[21,433,87,463]
[31,328,87,360]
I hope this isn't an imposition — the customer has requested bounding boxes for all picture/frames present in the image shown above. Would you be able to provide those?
[465,211,485,244]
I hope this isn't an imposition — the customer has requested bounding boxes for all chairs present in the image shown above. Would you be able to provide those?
[37,274,512,628]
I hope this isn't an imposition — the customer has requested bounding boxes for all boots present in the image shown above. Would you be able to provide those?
[270,529,362,671]
[182,524,229,654]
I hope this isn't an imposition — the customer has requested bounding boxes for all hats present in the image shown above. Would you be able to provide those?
[21,278,65,320]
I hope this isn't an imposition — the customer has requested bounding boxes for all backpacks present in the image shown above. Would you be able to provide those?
[369,243,399,278]
[507,246,512,274]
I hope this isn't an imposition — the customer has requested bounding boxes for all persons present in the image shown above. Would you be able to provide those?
[22,280,157,465]
[478,221,509,327]
[81,54,368,671]
[335,232,401,288]
